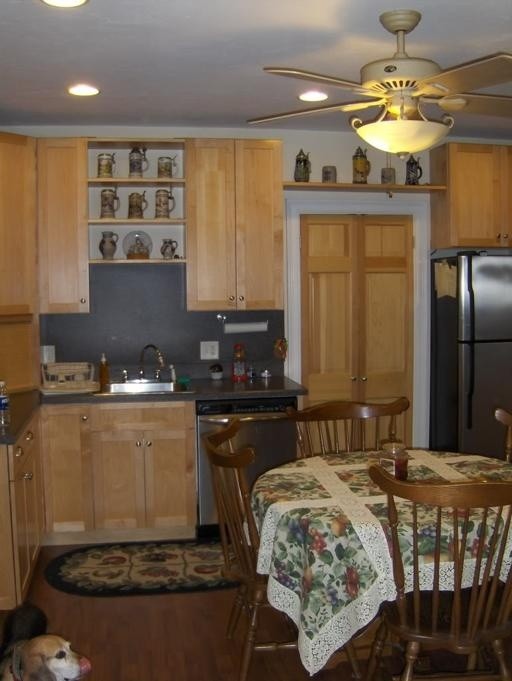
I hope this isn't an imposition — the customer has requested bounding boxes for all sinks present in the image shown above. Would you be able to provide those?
[90,381,197,396]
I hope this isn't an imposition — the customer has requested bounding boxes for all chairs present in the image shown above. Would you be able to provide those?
[365,465,512,681]
[494,408,512,462]
[204,420,364,681]
[287,397,410,458]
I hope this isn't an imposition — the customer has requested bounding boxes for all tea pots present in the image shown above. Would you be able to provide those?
[98,230,119,260]
[159,237,178,259]
[294,148,312,182]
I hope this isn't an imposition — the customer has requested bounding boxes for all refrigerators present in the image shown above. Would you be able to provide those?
[431,246,512,458]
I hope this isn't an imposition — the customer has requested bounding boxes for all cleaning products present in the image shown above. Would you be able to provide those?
[99,353,109,386]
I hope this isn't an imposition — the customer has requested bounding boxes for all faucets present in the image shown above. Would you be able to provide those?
[139,344,165,380]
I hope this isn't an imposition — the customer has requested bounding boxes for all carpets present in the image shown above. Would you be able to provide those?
[44,542,239,598]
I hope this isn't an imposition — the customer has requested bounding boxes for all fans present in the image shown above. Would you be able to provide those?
[246,11,512,127]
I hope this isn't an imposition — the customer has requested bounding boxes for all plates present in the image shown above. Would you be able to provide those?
[122,231,153,253]
[38,381,101,395]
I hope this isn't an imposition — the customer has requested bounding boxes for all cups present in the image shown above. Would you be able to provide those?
[381,168,395,184]
[210,364,223,379]
[352,146,370,184]
[158,156,178,178]
[97,153,114,177]
[322,166,336,183]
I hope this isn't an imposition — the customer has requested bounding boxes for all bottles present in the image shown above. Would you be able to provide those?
[405,154,422,185]
[247,360,255,379]
[156,185,175,219]
[101,190,120,218]
[0,380,10,437]
[378,444,409,481]
[232,344,246,380]
[129,148,148,178]
[128,191,148,219]
[98,354,108,393]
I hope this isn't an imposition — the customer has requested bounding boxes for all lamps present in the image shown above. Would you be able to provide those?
[349,100,454,160]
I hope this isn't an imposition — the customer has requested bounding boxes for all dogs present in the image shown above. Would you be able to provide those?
[0,634,92,681]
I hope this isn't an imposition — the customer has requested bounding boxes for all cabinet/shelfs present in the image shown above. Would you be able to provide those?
[38,403,91,545]
[87,137,185,265]
[184,138,284,312]
[430,142,512,250]
[91,403,197,545]
[0,131,37,316]
[0,417,38,611]
[38,137,90,313]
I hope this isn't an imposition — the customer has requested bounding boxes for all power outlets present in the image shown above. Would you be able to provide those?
[200,341,219,360]
